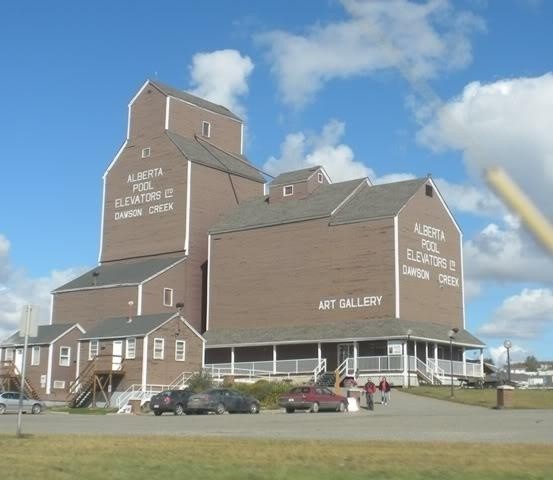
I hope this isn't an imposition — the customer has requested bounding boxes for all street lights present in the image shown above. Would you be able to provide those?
[504,336,515,387]
[448,329,460,399]
[406,327,415,389]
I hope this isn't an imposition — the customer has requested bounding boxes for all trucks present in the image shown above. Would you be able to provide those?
[458,372,509,389]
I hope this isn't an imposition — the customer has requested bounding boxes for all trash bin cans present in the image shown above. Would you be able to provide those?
[129,400,141,415]
[347,389,360,410]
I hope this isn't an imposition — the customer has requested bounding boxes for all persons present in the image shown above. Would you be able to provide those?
[362,377,376,411]
[354,367,359,385]
[379,376,391,406]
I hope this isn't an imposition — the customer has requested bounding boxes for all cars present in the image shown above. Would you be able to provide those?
[277,384,351,414]
[149,389,210,417]
[187,387,263,416]
[0,390,48,415]
